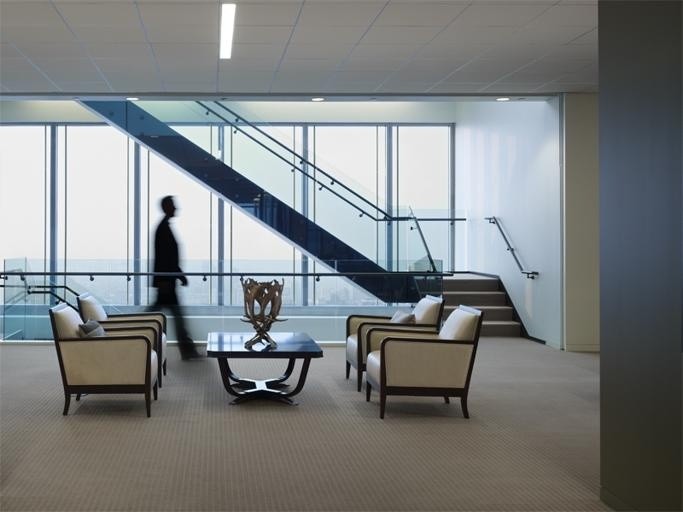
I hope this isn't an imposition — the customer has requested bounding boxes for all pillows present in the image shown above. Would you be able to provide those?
[391,311,414,324]
[79,320,108,338]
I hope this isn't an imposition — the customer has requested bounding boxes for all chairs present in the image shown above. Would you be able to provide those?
[346,294,446,392]
[77,293,167,388]
[366,305,483,419]
[48,302,158,418]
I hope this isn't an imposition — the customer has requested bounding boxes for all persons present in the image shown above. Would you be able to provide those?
[142,194,206,361]
